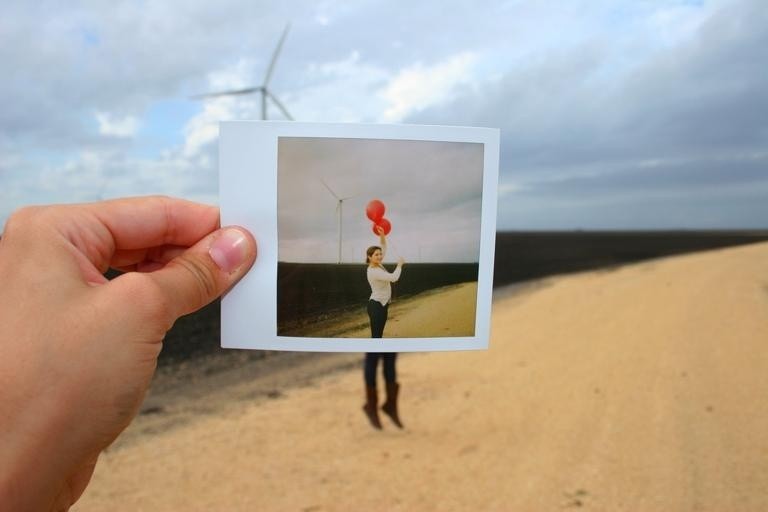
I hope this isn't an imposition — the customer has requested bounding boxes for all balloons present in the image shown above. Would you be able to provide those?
[366,200,386,223]
[372,219,391,236]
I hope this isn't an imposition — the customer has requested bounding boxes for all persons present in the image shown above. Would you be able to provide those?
[365,225,404,338]
[0,195,257,512]
[362,352,402,430]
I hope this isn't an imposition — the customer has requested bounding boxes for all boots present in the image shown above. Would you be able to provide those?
[364,384,401,429]
